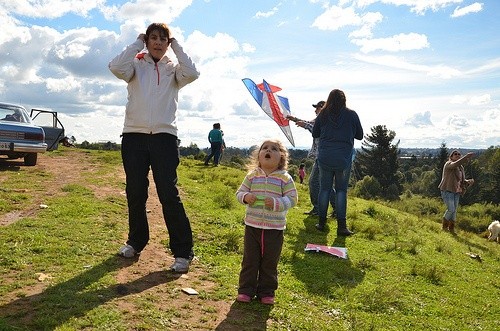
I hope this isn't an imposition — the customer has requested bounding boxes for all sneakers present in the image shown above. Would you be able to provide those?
[119,244,138,257]
[170,257,190,272]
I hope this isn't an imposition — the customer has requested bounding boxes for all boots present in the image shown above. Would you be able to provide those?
[448,219,457,235]
[315,218,327,232]
[442,217,449,231]
[337,218,354,237]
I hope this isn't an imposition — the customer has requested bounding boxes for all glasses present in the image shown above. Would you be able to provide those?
[452,153,457,155]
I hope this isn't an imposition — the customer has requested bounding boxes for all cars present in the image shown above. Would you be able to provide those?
[0,102,65,166]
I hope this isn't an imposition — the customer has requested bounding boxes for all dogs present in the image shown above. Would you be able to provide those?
[487,220,500,243]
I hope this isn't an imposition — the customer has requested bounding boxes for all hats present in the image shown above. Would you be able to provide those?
[313,101,326,108]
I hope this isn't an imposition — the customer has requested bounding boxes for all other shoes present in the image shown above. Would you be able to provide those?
[204,163,209,166]
[237,294,251,303]
[328,210,336,217]
[304,209,319,216]
[213,164,218,167]
[258,296,274,304]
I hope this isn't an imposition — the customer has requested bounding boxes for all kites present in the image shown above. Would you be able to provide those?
[243,77,295,146]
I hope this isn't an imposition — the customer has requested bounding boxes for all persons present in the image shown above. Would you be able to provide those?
[286,102,337,219]
[312,89,364,235]
[437,150,475,234]
[204,123,226,167]
[237,139,298,304]
[107,23,202,271]
[297,163,306,183]
